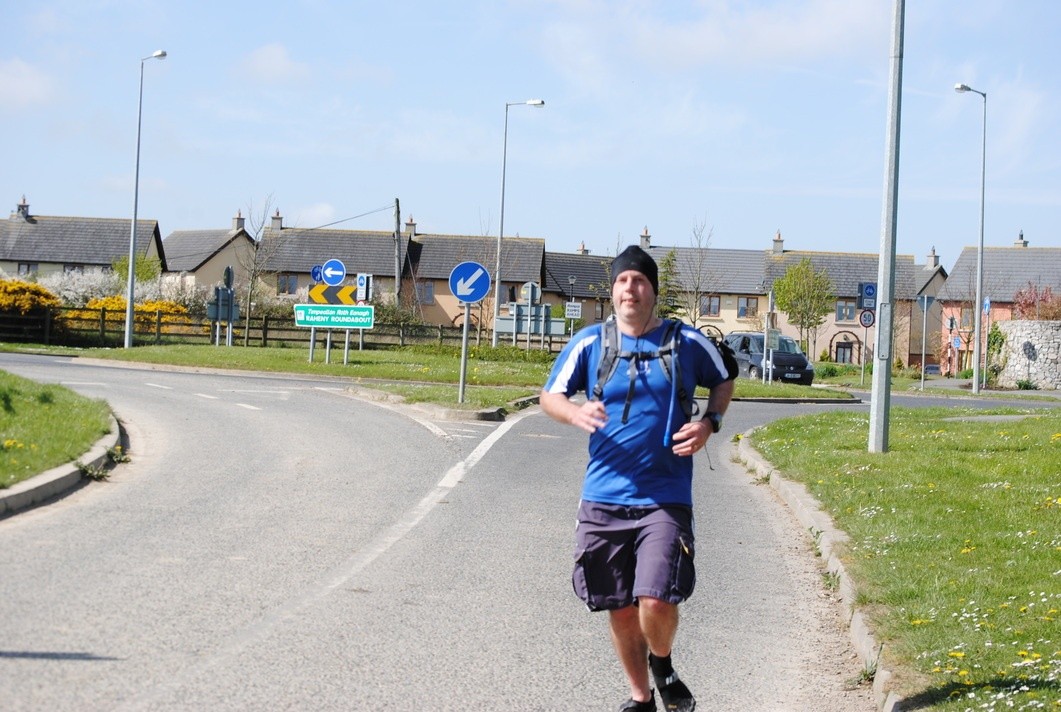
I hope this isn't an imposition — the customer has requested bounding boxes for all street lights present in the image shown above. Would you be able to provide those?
[123,48,168,348]
[951,81,990,393]
[567,275,577,336]
[492,99,548,347]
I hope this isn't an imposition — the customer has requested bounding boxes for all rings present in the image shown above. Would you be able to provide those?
[695,445,698,450]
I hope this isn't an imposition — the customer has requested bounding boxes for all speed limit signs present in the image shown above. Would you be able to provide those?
[859,310,875,327]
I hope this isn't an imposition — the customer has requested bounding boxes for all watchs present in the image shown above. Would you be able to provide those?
[702,411,723,434]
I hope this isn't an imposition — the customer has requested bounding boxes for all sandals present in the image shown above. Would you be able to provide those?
[653,669,696,712]
[619,688,657,712]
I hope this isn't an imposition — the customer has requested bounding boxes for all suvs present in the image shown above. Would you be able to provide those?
[722,330,816,387]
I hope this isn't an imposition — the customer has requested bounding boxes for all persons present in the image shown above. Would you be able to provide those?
[540,245,739,712]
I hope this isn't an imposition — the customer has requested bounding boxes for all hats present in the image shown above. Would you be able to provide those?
[609,246,658,294]
